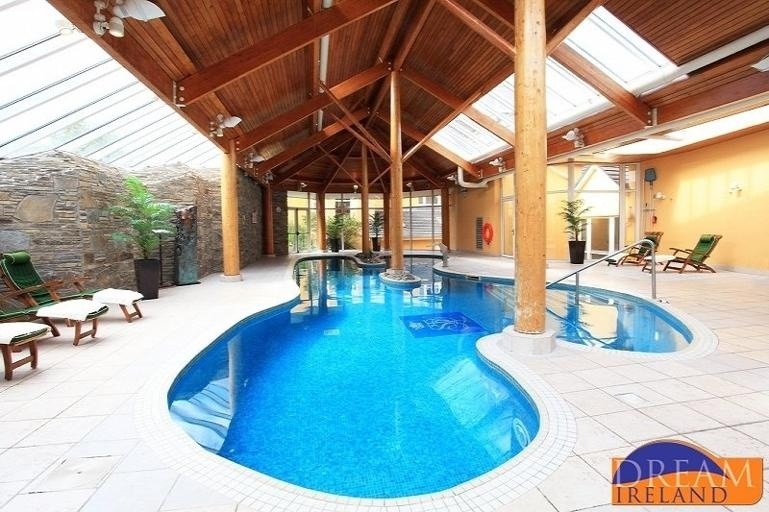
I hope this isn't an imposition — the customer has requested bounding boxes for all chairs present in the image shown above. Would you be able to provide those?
[1,249,110,344]
[607,231,723,273]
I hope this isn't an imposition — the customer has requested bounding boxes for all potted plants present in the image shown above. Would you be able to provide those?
[326,214,345,252]
[107,174,182,298]
[369,212,385,251]
[559,198,591,264]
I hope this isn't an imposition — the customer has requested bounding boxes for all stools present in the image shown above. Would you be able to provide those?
[0,320,48,380]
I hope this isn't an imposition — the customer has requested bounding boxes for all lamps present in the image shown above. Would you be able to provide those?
[210,113,242,137]
[750,51,769,74]
[562,127,584,149]
[244,151,265,168]
[489,156,505,173]
[93,0,168,38]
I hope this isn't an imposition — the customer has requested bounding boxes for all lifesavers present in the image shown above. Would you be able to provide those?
[482,223,493,243]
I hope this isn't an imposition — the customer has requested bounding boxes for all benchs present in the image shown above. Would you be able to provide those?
[57,279,144,324]
